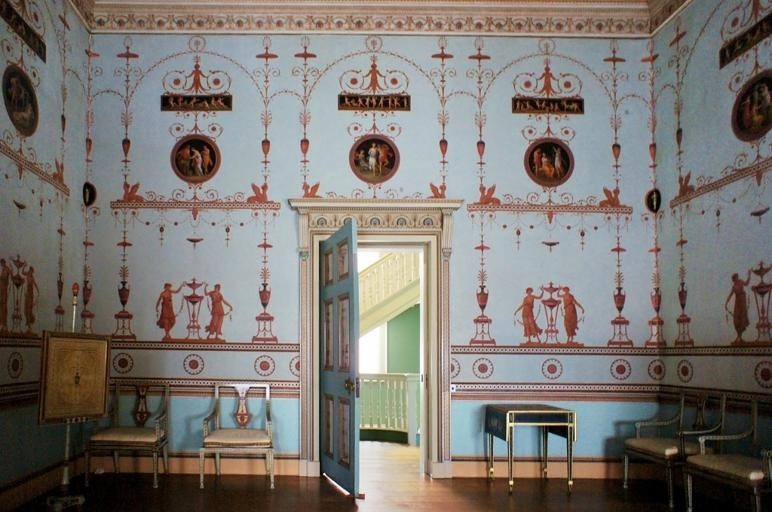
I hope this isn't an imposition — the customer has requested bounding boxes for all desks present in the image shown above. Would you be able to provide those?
[484,404,577,496]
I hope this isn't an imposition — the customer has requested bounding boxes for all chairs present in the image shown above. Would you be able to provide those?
[622,389,772,512]
[82,382,276,491]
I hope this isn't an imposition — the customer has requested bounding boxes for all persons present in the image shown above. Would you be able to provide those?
[741,83,770,133]
[357,142,394,174]
[722,268,752,342]
[154,281,183,337]
[20,263,41,332]
[533,147,565,180]
[9,77,26,110]
[513,286,545,342]
[0,260,13,329]
[202,283,234,338]
[178,143,213,177]
[556,286,586,341]
